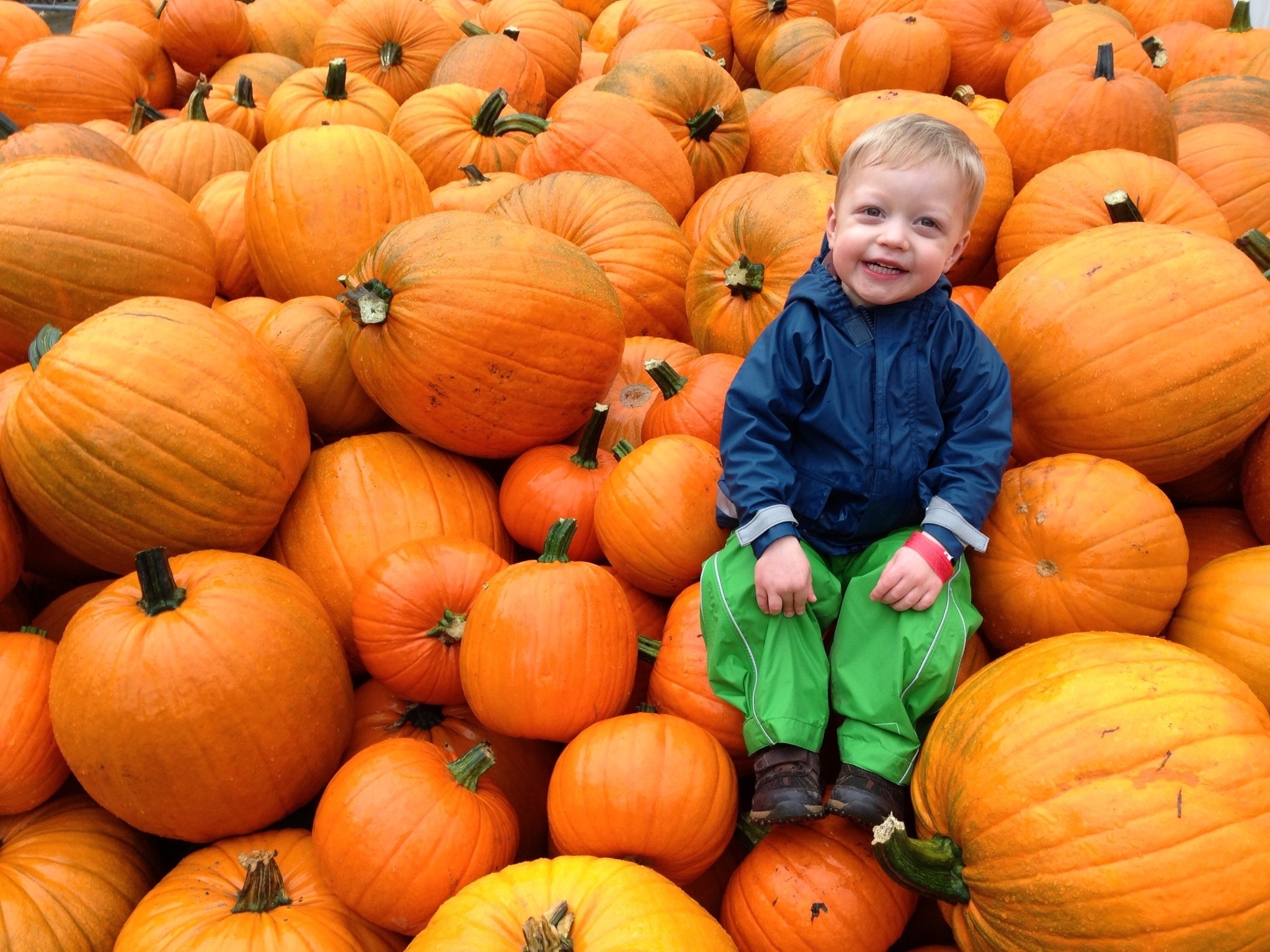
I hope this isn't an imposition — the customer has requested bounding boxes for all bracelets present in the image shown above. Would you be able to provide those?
[902,531,954,584]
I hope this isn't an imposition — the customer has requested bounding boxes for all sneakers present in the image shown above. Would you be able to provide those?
[748,744,826,823]
[826,764,897,833]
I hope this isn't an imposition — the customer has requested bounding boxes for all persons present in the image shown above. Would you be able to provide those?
[700,112,1013,825]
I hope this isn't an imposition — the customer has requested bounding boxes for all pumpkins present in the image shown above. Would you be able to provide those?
[0,0,1270,951]
